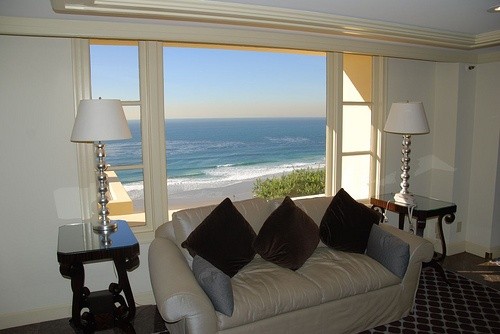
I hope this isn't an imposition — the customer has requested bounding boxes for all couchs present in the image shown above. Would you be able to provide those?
[148,195,434,334]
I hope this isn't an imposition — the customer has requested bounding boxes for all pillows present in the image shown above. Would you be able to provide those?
[248,195,321,271]
[319,188,382,254]
[181,198,257,279]
[192,255,234,317]
[366,223,409,279]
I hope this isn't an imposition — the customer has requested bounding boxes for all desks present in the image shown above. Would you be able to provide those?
[369,193,457,286]
[57,220,140,334]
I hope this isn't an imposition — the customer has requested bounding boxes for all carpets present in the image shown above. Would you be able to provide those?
[153,266,500,334]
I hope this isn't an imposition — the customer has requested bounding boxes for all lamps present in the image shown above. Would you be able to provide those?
[70,98,132,230]
[383,100,430,203]
